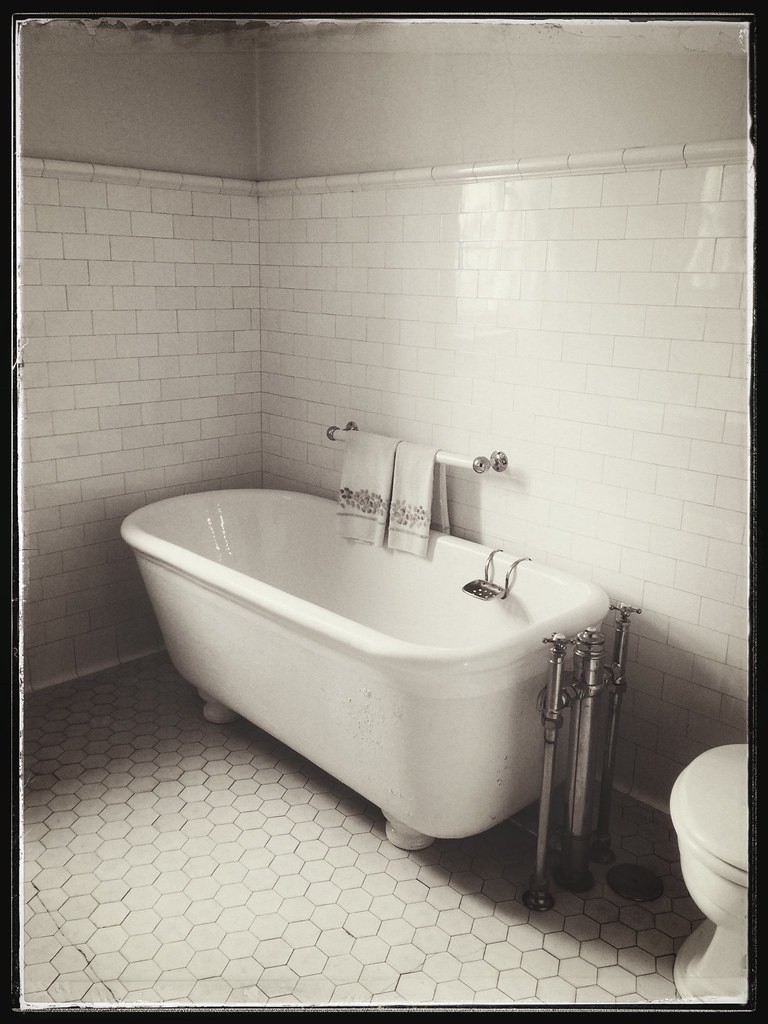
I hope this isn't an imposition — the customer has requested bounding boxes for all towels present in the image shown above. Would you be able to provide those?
[335,430,402,548]
[388,442,450,557]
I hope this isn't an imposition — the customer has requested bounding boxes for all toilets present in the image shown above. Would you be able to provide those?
[669,743,749,1002]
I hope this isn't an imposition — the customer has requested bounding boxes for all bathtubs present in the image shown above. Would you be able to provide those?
[118,488,611,837]
